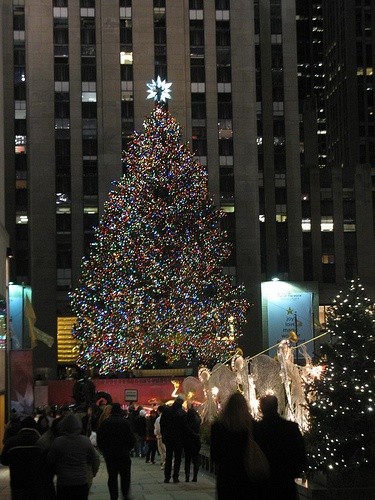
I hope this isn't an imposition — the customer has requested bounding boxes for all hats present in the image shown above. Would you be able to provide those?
[110,402,124,415]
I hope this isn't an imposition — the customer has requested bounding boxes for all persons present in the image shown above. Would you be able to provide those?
[182,408,201,481]
[160,397,187,483]
[97,404,134,500]
[211,393,256,499]
[0,417,58,500]
[4,404,173,470]
[255,395,305,500]
[52,414,100,499]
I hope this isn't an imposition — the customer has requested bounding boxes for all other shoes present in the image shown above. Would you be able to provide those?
[185,478,189,482]
[164,476,171,483]
[172,474,179,483]
[192,476,198,482]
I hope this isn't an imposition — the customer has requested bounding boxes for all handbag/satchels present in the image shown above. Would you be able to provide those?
[245,438,273,487]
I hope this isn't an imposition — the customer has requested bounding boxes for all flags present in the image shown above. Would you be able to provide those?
[23,294,36,349]
[267,290,314,359]
